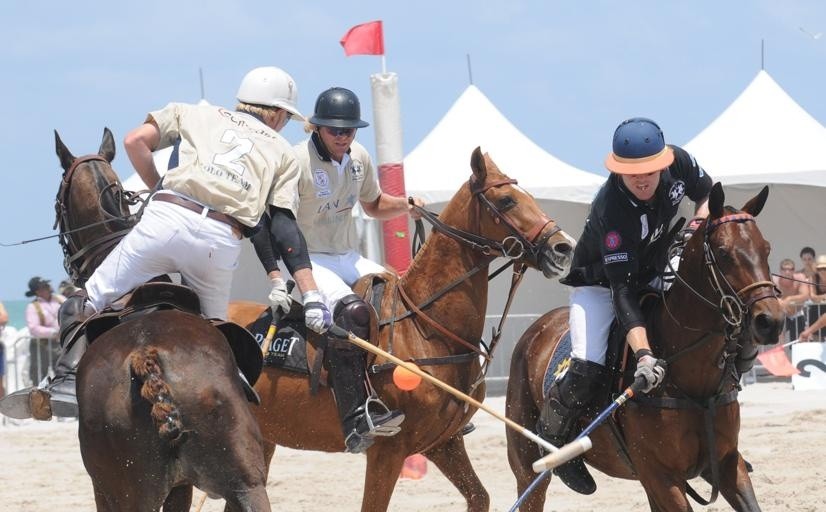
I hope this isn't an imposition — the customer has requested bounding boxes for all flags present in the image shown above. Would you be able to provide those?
[756,346,801,378]
[340,22,384,56]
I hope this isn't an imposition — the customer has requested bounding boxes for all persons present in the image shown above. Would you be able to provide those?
[533,119,753,494]
[26,276,69,386]
[799,313,826,342]
[245,88,474,452]
[0,65,334,419]
[769,259,810,348]
[808,255,826,341]
[1,301,9,396]
[796,247,816,279]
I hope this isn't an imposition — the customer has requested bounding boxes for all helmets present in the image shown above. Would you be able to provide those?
[604,118,676,176]
[309,86,371,129]
[237,64,307,123]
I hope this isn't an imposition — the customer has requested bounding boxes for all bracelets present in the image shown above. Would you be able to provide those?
[636,348,655,360]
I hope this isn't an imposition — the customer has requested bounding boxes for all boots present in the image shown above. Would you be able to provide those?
[462,422,476,436]
[323,294,402,455]
[536,356,608,496]
[0,292,98,420]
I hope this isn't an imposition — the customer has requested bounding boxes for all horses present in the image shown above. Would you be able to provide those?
[54,127,271,512]
[505,182,785,512]
[207,147,578,512]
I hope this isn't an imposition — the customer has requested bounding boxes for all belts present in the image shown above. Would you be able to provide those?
[153,192,244,232]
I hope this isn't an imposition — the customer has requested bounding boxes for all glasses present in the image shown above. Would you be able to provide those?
[780,266,795,273]
[326,126,358,136]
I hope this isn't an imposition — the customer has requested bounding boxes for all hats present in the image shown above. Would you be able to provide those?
[816,254,826,268]
[25,276,53,296]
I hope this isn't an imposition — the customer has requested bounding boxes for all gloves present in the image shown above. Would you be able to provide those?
[302,289,332,335]
[269,277,293,320]
[673,217,705,245]
[633,354,668,393]
